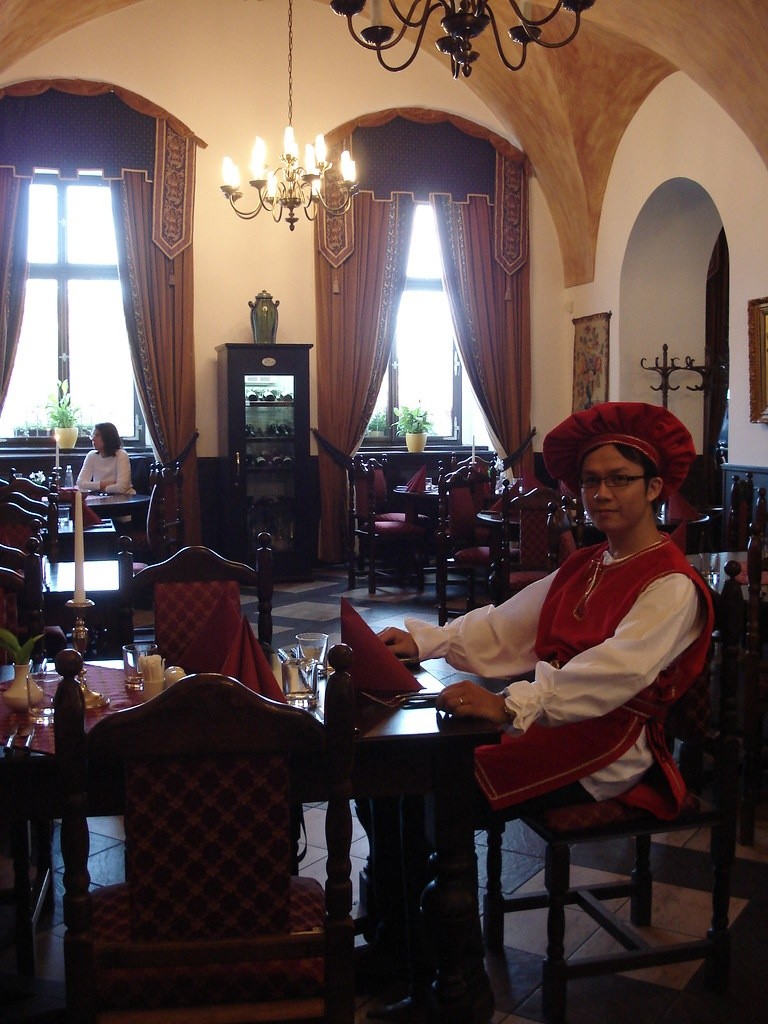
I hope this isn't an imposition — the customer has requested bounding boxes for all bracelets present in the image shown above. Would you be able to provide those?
[502,690,516,725]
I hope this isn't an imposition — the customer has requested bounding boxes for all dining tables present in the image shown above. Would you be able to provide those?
[0,661,502,753]
[39,493,149,594]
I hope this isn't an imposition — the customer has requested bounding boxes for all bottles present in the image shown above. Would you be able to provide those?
[65,465,74,487]
[245,388,295,534]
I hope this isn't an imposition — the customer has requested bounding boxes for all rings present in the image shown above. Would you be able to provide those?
[459,697,464,706]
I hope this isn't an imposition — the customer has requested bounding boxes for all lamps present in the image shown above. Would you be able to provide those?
[330,0,596,80]
[220,0,359,231]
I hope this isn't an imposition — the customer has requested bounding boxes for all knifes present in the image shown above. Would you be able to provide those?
[24,724,35,749]
[3,723,19,752]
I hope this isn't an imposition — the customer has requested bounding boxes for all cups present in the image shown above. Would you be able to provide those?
[700,552,720,579]
[141,679,166,702]
[424,478,432,490]
[122,643,157,690]
[295,632,330,677]
[282,658,318,708]
[26,671,64,724]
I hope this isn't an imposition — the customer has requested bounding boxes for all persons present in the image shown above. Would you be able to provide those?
[76,423,137,529]
[353,403,715,1024]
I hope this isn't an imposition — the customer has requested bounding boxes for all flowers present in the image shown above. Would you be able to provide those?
[493,456,506,472]
[28,469,45,485]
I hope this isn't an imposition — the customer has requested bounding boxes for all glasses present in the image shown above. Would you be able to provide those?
[575,473,647,489]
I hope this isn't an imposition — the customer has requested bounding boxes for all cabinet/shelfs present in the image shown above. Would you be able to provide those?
[214,343,314,579]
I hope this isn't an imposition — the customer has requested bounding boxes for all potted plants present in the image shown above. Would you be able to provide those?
[394,403,433,451]
[46,380,79,448]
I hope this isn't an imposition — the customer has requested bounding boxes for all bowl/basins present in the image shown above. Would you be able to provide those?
[57,489,91,501]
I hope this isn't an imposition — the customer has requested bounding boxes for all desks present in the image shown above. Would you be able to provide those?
[394,480,768,607]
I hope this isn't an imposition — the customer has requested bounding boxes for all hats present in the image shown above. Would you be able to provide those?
[542,402,697,498]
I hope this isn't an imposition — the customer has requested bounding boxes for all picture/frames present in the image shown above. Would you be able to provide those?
[746,297,768,422]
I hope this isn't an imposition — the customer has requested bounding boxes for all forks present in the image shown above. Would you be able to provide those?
[359,690,440,708]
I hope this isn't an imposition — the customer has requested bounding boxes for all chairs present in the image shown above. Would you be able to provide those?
[485,561,742,1023]
[119,533,276,659]
[0,461,184,561]
[354,449,578,625]
[0,537,46,661]
[688,473,768,662]
[53,645,354,1023]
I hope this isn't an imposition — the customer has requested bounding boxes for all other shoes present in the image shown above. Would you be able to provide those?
[365,973,496,1024]
